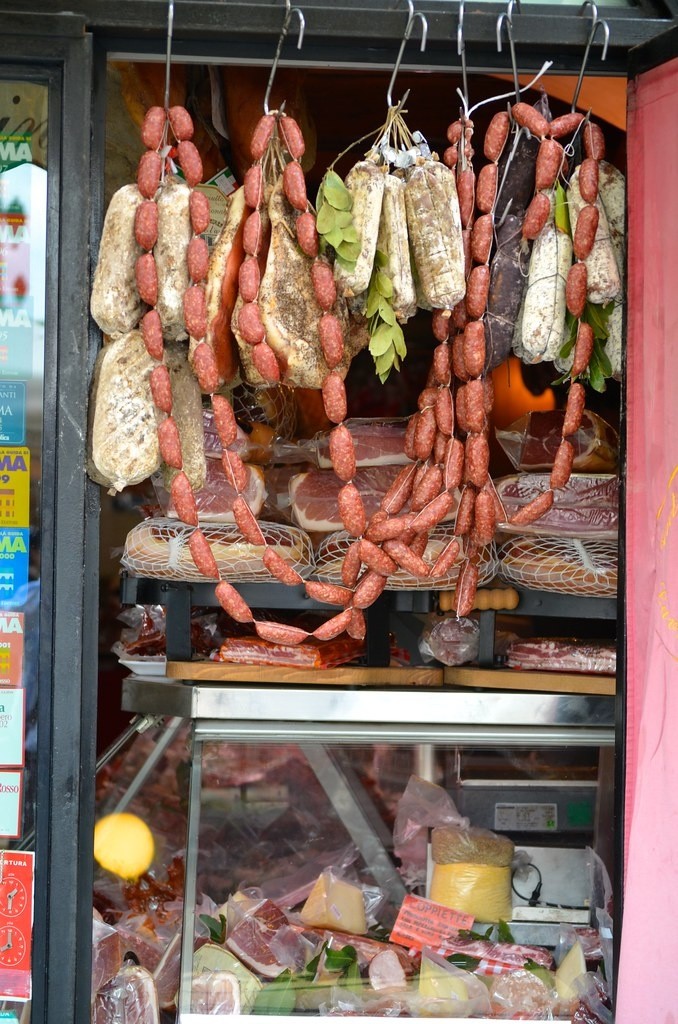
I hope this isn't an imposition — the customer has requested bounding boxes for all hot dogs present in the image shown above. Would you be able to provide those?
[134,75,603,653]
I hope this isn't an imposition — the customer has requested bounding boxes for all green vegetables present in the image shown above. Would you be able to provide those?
[202,902,557,1015]
